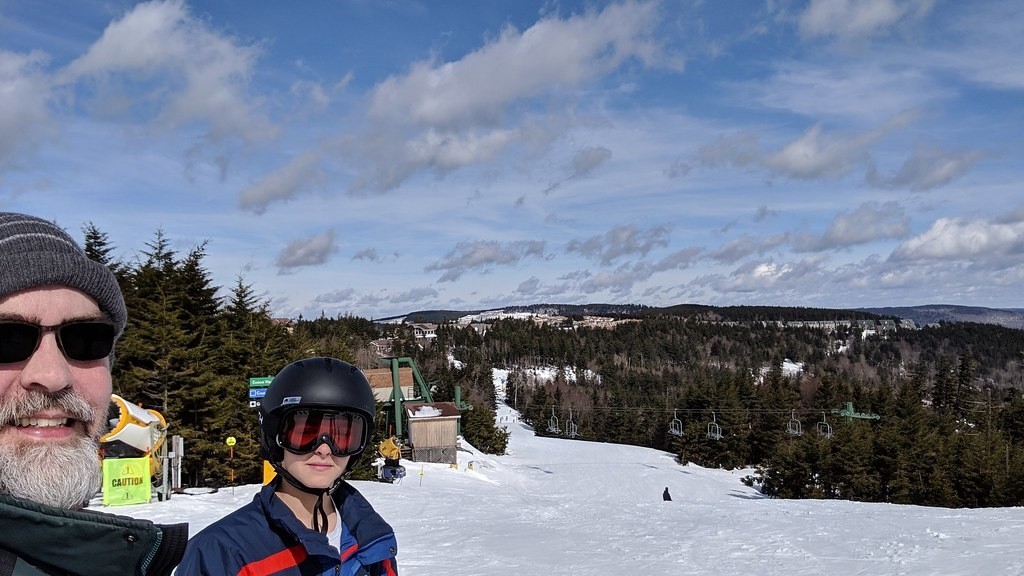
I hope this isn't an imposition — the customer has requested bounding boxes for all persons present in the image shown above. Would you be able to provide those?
[174,356,398,576]
[663,486,672,501]
[0,211,189,576]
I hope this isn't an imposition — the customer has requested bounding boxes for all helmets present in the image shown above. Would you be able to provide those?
[256,357,375,429]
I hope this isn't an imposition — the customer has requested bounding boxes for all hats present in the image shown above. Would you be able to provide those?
[0,211,127,344]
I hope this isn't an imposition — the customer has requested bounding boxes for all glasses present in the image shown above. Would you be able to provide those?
[0,319,117,365]
[266,406,368,456]
